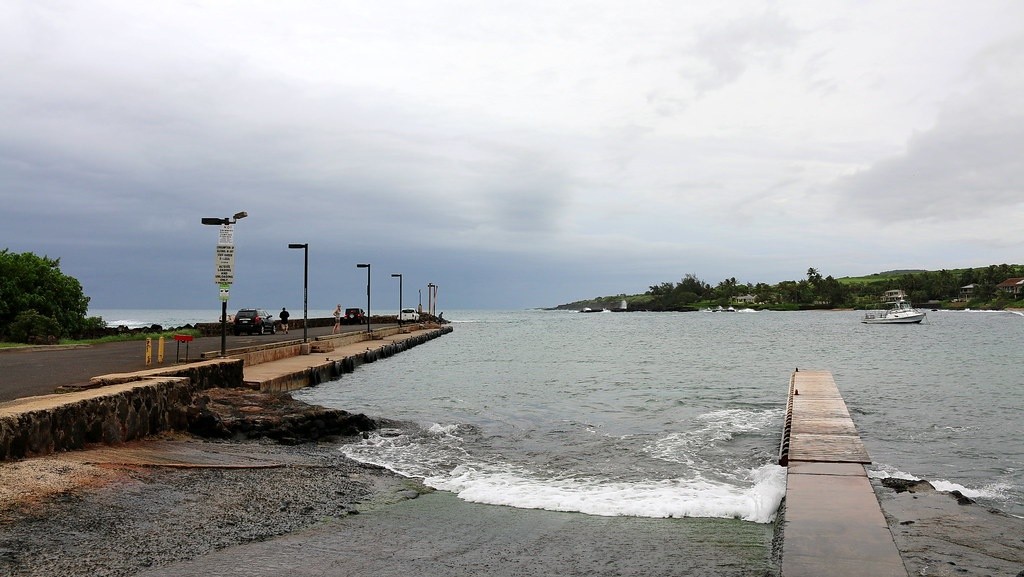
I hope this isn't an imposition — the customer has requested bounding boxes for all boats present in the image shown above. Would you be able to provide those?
[860,301,926,324]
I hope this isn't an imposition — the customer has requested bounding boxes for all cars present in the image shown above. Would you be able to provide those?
[396,308,420,325]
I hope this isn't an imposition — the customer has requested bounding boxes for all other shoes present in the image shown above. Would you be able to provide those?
[283,330,289,334]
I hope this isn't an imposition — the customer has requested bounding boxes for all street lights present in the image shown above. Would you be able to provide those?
[391,273,402,327]
[201,211,248,357]
[428,284,436,317]
[289,243,309,343]
[357,263,371,333]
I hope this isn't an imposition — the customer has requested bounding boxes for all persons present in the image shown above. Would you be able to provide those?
[279,307,289,334]
[331,304,341,335]
[437,312,443,324]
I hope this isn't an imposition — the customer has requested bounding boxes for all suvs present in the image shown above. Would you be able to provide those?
[342,308,367,325]
[233,308,277,336]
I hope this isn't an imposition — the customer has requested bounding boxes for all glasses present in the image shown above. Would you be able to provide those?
[338,306,341,307]
